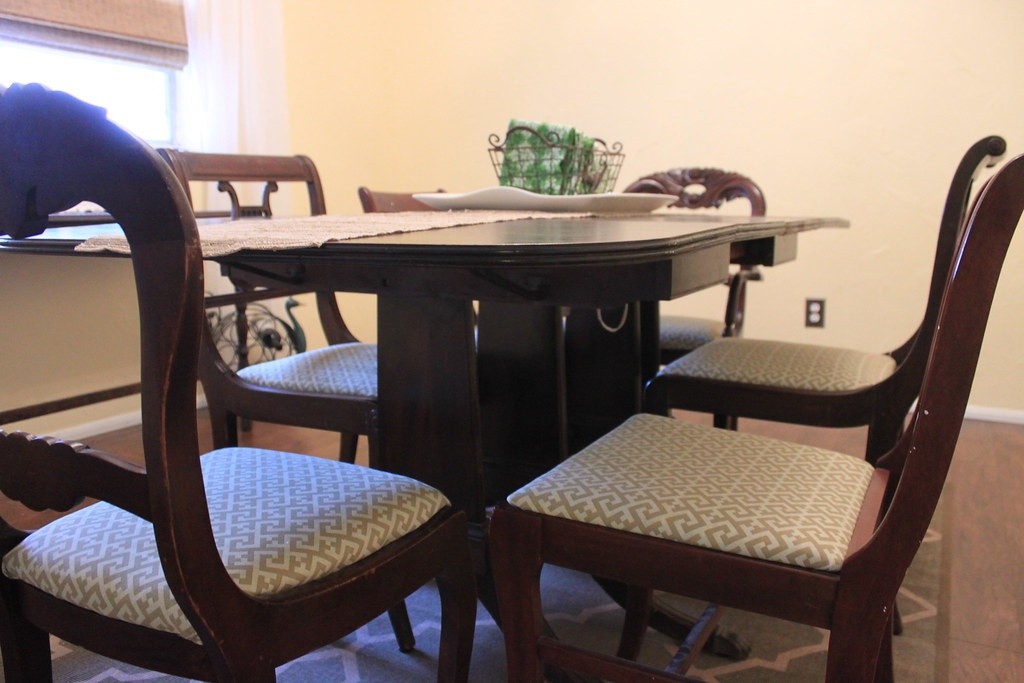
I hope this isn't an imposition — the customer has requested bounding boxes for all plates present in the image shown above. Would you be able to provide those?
[412,186,680,210]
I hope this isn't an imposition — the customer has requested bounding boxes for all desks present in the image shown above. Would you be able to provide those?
[0,210,851,683]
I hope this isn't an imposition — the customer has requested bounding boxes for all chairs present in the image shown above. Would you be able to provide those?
[641,133,1010,466]
[617,167,767,430]
[485,152,1023,683]
[145,146,377,464]
[0,80,477,683]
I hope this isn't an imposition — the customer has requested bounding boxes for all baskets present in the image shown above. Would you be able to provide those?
[488,127,624,195]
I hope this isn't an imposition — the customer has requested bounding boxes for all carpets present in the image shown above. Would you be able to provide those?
[0,464,959,683]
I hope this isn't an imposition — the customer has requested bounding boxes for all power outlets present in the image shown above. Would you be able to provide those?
[805,297,825,329]
